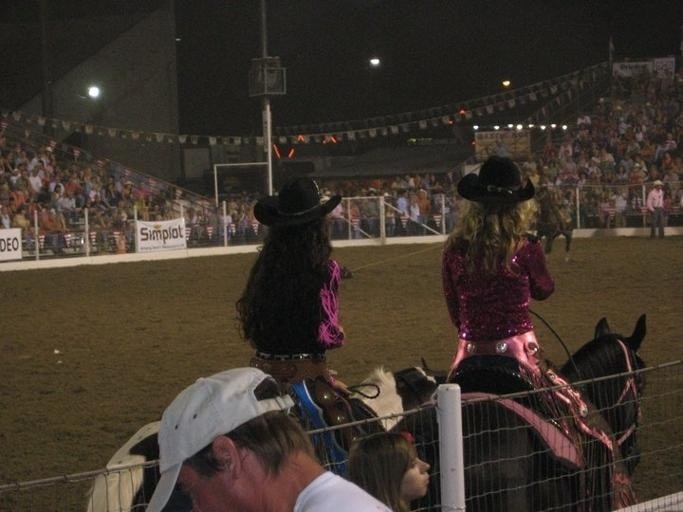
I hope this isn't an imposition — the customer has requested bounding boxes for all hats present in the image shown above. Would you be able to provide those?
[253,177,342,226]
[147,368,295,511]
[457,156,537,204]
[652,180,664,187]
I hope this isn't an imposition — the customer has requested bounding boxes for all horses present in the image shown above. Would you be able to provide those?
[535,185,575,262]
[82,352,455,512]
[384,314,653,511]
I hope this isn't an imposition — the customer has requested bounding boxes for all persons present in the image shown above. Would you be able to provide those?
[347,432,431,512]
[445,154,638,512]
[143,367,394,512]
[234,174,359,456]
